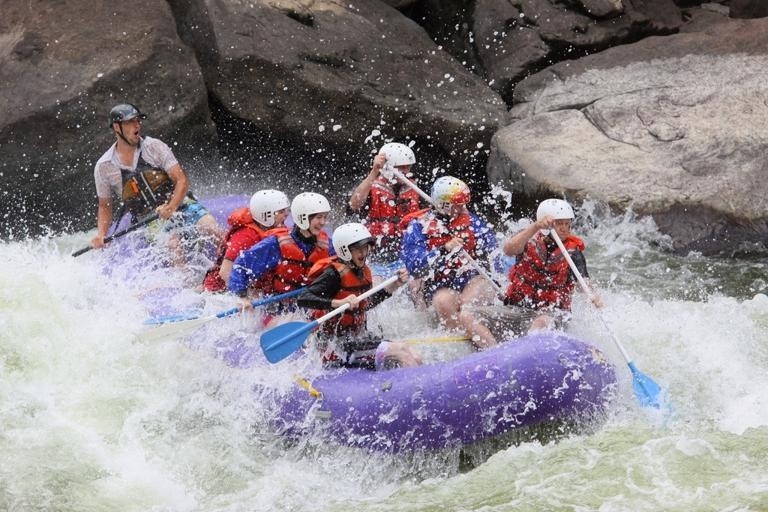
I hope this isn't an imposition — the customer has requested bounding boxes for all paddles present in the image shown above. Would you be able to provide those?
[551,228,668,410]
[328,273,330,274]
[143,287,310,344]
[260,276,399,364]
[386,162,517,270]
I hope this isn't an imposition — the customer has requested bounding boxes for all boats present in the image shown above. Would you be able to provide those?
[98,195,618,453]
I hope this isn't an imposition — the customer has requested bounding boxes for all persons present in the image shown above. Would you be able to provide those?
[399,176,503,331]
[229,191,337,318]
[490,217,535,283]
[91,104,227,266]
[294,222,410,370]
[204,188,292,293]
[457,197,604,351]
[346,142,431,264]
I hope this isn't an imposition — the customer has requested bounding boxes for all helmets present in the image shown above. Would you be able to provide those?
[249,189,290,228]
[290,191,331,230]
[332,223,376,261]
[536,198,575,235]
[109,103,141,128]
[377,141,417,179]
[431,175,471,216]
